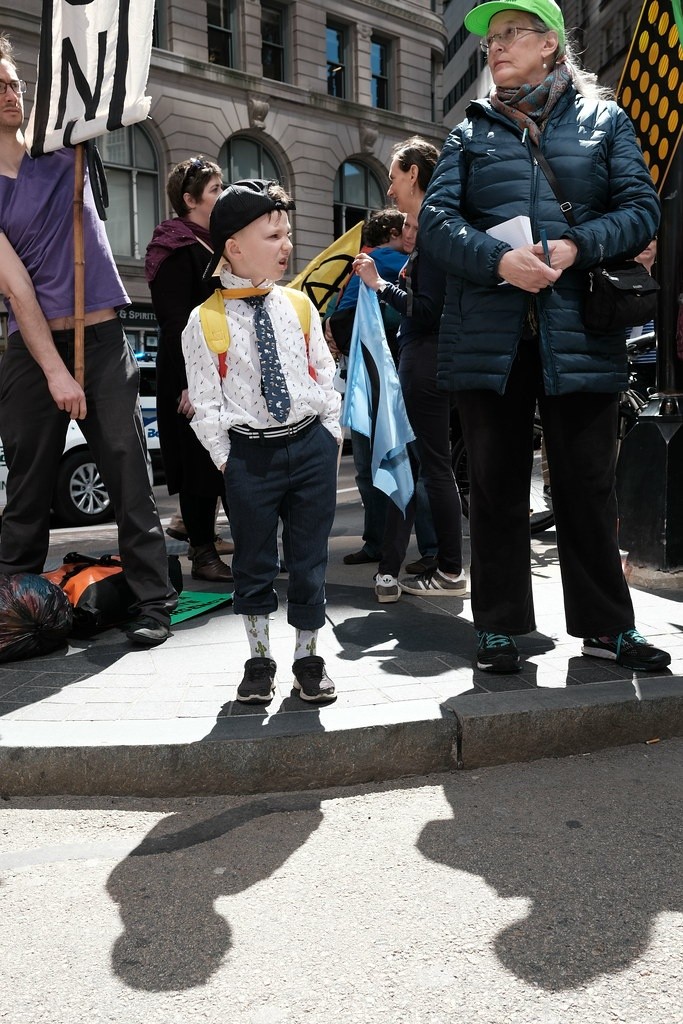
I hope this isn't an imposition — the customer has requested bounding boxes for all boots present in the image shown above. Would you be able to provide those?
[188,543,234,581]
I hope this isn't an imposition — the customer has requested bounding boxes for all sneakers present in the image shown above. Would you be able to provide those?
[291,655,337,701]
[582,627,671,670]
[237,657,278,702]
[398,565,467,597]
[374,573,401,602]
[475,629,522,673]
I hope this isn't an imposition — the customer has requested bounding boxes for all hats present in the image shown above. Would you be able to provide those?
[464,0,566,57]
[203,178,296,278]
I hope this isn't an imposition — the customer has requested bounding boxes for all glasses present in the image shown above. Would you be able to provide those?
[184,158,205,186]
[480,26,547,52]
[0,80,27,94]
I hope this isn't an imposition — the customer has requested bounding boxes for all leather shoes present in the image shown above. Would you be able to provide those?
[165,528,190,543]
[406,554,439,574]
[343,545,377,565]
[187,536,235,555]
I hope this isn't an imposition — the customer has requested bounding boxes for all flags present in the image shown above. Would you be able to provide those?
[285,222,363,320]
[343,275,414,519]
[24,0,151,156]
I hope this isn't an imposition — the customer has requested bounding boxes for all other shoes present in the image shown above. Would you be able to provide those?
[529,509,556,535]
[543,485,552,500]
[126,613,169,644]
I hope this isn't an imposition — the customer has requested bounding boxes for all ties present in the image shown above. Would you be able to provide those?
[237,292,291,423]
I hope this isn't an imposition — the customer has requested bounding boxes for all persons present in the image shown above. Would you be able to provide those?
[145,158,233,556]
[0,38,178,644]
[182,177,342,705]
[416,0,683,673]
[354,138,468,597]
[322,207,446,572]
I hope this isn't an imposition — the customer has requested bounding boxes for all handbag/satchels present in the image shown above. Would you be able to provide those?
[0,573,73,665]
[39,547,184,639]
[581,260,660,333]
